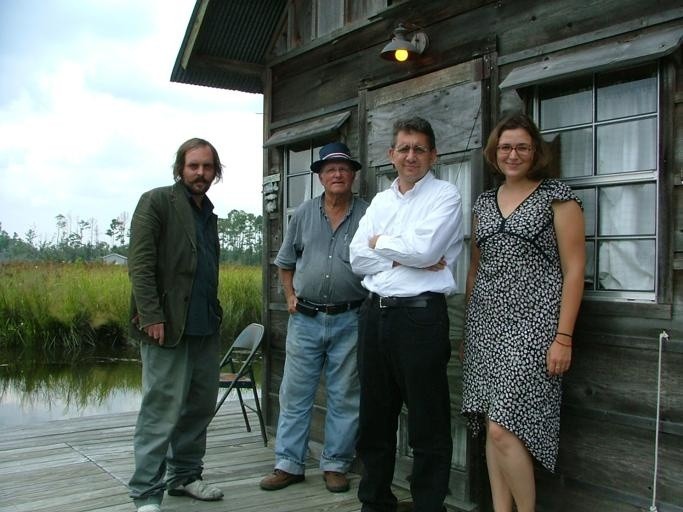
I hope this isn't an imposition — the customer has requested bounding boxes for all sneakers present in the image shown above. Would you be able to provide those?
[323,469,349,494]
[259,466,305,491]
[166,477,223,501]
[136,495,161,512]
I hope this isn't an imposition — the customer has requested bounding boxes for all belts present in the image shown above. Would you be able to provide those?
[295,297,363,316]
[366,289,441,309]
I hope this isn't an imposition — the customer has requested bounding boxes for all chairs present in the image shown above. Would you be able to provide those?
[208,323,268,446]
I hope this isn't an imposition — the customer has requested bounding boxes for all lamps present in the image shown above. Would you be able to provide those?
[379,23,427,62]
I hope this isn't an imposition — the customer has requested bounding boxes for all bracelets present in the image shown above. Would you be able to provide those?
[557,332,574,337]
[555,339,572,347]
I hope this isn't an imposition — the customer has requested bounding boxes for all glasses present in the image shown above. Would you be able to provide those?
[395,145,431,154]
[494,143,535,154]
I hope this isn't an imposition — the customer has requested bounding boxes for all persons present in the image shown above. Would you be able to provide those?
[349,115,463,512]
[260,143,371,490]
[460,114,585,512]
[128,139,226,512]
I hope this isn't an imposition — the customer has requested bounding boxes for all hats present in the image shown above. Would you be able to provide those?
[309,141,361,173]
